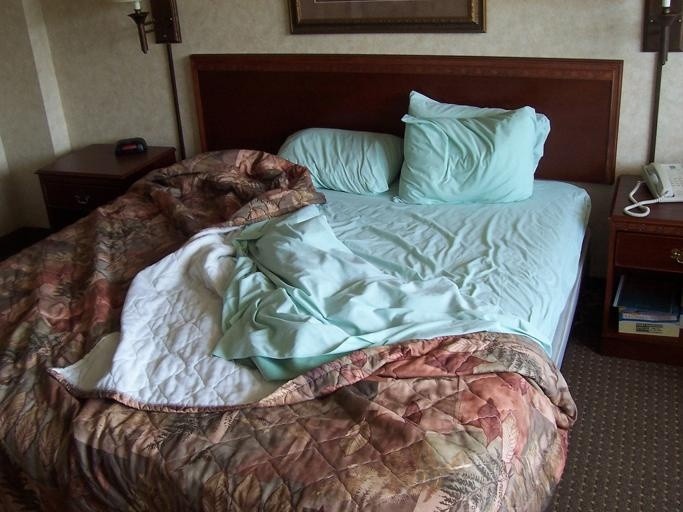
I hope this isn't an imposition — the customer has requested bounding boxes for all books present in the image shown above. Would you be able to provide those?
[613,275,681,323]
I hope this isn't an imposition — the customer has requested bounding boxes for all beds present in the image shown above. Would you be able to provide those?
[1,57,624,510]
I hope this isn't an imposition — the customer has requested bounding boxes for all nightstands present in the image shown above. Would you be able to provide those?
[601,174,683,360]
[33,136,175,235]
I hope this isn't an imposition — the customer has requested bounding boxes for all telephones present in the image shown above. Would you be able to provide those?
[641,163,683,203]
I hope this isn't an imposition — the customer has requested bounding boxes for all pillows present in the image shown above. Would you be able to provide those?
[278,127,403,194]
[393,106,537,203]
[405,91,552,174]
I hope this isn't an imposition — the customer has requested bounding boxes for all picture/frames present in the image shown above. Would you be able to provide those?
[286,0,489,35]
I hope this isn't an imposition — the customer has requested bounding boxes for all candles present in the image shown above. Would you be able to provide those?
[661,0,671,7]
[133,0,142,10]
